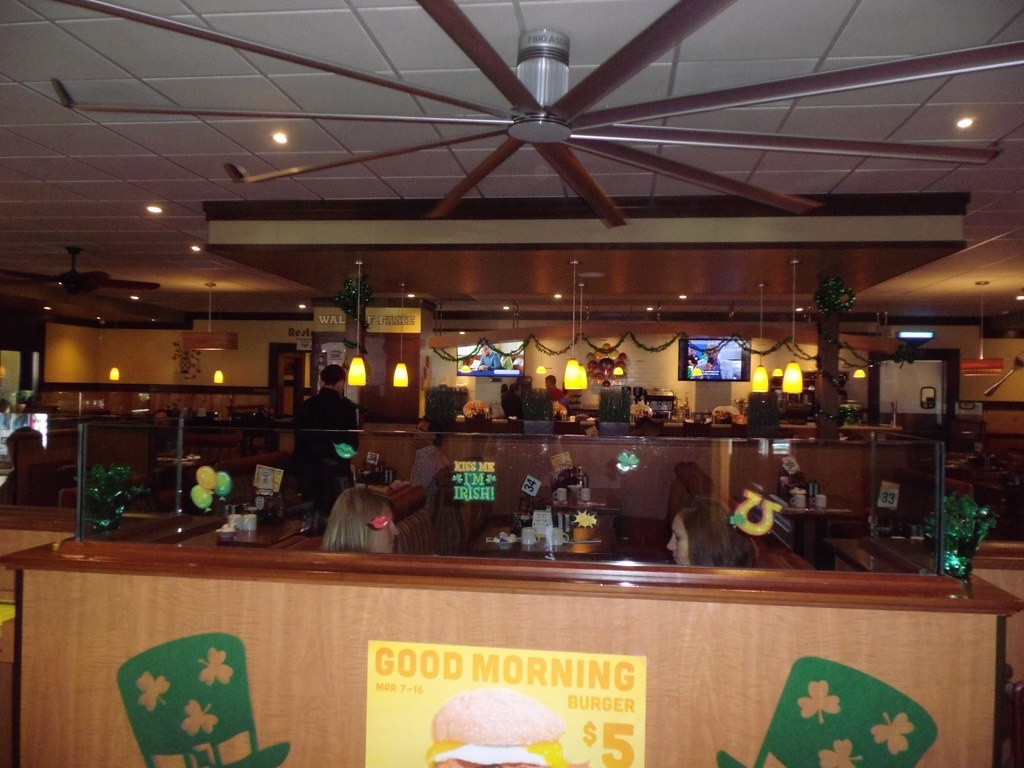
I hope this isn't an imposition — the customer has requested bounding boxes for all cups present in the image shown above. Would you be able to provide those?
[551,527,569,545]
[242,514,256,531]
[553,488,567,505]
[197,408,206,417]
[810,494,827,508]
[790,495,807,508]
[228,515,242,531]
[577,488,590,502]
[521,527,540,545]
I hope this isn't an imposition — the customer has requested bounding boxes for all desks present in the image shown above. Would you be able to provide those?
[472,525,623,560]
[824,538,901,573]
[177,515,314,547]
[0,529,77,768]
[754,492,852,558]
[537,485,624,525]
[158,458,218,466]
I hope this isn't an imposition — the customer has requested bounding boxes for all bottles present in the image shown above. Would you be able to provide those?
[389,467,394,484]
[583,474,588,488]
[578,467,584,484]
[384,467,389,483]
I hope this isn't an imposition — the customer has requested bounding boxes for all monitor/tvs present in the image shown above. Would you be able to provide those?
[457,342,525,378]
[677,338,751,381]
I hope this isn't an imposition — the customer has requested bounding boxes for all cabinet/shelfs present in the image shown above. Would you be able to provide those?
[771,369,848,401]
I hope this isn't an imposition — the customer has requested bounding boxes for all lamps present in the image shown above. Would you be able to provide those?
[348,260,409,389]
[749,261,803,394]
[852,366,866,378]
[535,260,624,390]
[182,282,238,383]
[109,320,120,381]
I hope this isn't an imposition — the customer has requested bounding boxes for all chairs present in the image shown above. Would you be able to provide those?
[636,416,750,437]
[465,417,580,433]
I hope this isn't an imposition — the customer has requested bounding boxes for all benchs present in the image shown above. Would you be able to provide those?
[16,435,521,556]
[865,466,974,536]
[612,460,819,571]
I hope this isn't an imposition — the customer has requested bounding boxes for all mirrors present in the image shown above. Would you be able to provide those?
[920,387,937,409]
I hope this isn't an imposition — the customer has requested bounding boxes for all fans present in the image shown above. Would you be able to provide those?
[0,247,160,296]
[51,0,1024,232]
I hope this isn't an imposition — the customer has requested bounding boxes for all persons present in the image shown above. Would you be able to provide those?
[478,345,500,372]
[688,343,735,380]
[666,495,759,567]
[322,487,399,554]
[1,427,53,508]
[282,365,360,517]
[410,416,453,488]
[151,410,172,424]
[628,403,662,437]
[501,375,576,418]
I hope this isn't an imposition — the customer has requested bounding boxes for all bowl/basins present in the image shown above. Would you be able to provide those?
[215,530,237,538]
[568,485,582,492]
[492,541,515,550]
